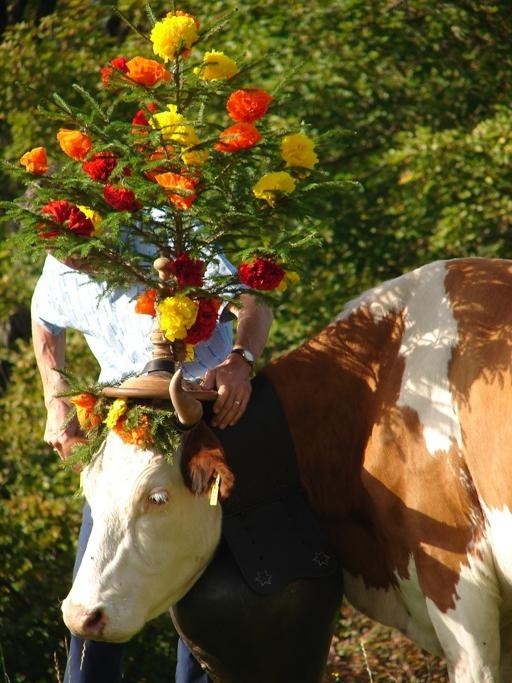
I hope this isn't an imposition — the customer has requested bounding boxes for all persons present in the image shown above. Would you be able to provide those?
[25,200,275,682]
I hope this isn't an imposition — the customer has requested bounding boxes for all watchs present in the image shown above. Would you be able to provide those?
[230,347,255,368]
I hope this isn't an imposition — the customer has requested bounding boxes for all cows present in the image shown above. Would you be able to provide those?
[52,256,512,683]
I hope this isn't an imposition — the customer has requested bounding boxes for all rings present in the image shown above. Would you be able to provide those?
[235,399,240,404]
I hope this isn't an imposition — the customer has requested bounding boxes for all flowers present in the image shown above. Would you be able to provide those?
[50,365,182,472]
[0,0,366,357]
